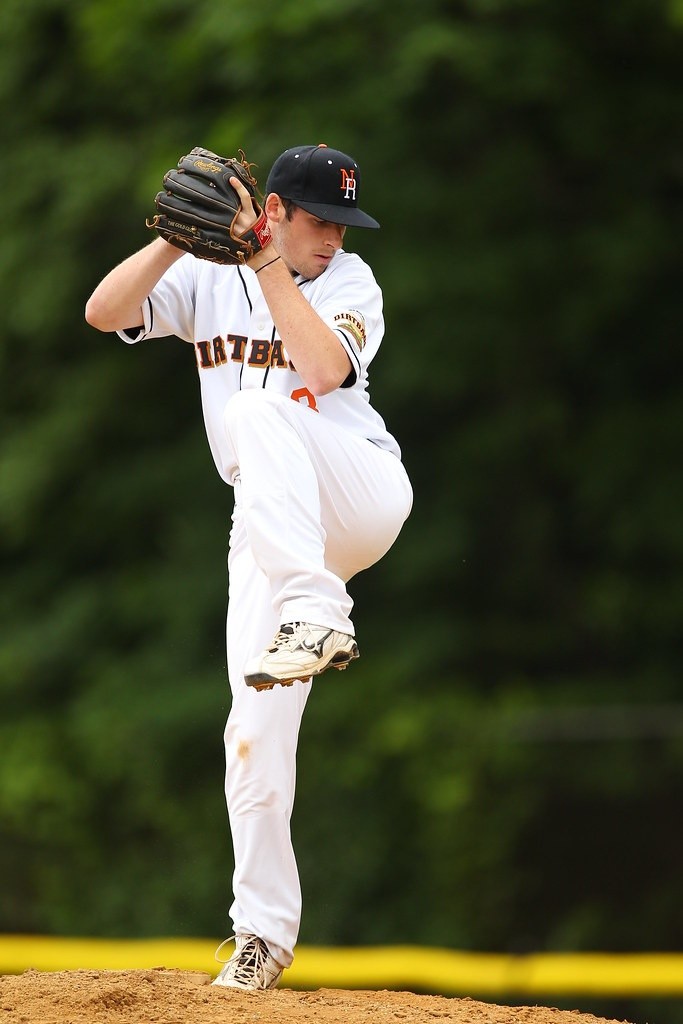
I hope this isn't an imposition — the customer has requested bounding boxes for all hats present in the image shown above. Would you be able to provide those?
[264,143,381,228]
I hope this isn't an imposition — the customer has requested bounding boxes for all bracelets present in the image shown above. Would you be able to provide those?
[254,255,281,274]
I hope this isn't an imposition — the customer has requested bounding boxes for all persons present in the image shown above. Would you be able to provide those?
[85,144,414,989]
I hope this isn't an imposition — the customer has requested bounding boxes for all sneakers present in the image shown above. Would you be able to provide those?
[242,623,361,692]
[211,934,284,990]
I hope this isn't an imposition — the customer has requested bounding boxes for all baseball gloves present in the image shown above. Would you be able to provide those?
[146,146,271,268]
